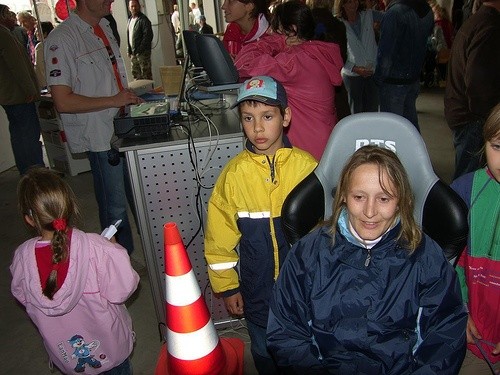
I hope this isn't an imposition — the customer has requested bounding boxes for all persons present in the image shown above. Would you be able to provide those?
[125,0,155,81]
[44,0,147,256]
[449,103,500,375]
[170,0,500,180]
[263,145,470,375]
[0,4,70,183]
[8,166,142,375]
[203,74,320,375]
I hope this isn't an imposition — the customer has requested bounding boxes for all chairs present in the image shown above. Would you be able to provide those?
[281,111,471,269]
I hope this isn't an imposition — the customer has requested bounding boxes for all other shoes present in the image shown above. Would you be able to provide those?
[56,172,66,177]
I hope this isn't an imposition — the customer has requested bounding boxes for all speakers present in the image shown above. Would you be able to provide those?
[197,33,239,85]
[182,30,204,70]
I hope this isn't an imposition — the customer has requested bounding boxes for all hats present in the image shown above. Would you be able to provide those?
[229,74,288,110]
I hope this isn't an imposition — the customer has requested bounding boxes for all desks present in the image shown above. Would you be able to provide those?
[118,87,247,342]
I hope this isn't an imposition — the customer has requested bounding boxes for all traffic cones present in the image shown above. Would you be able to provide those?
[153,222,245,375]
